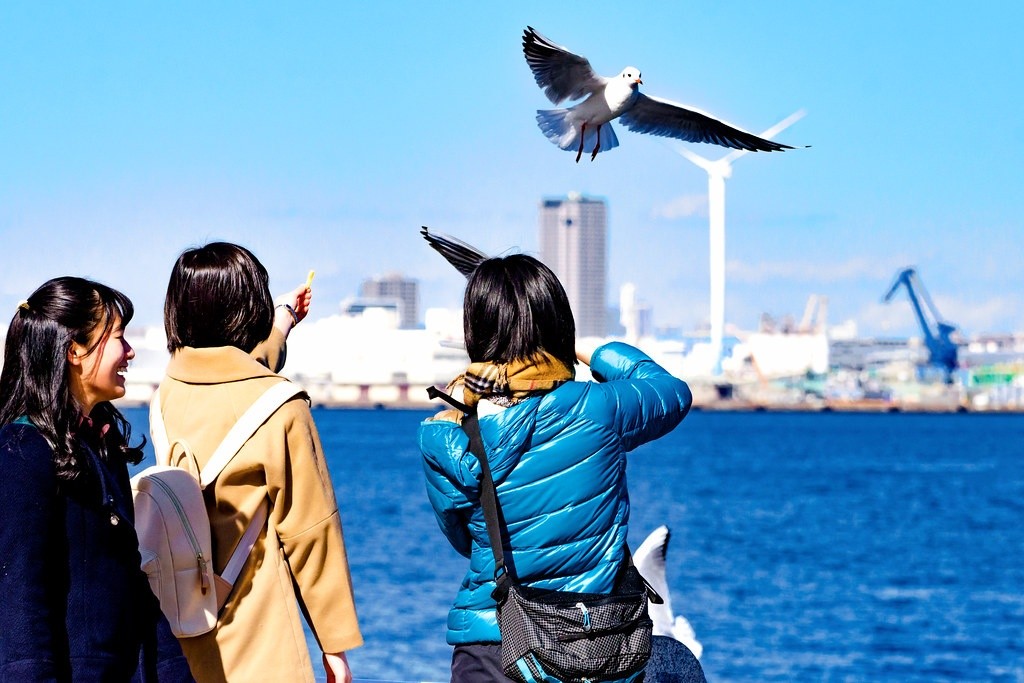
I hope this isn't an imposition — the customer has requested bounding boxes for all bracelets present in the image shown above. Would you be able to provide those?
[274,303,299,322]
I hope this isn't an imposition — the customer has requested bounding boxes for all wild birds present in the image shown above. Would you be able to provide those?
[632,526,703,662]
[521,24,814,164]
[421,225,491,281]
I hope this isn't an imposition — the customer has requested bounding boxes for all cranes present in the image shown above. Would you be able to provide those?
[883,268,968,385]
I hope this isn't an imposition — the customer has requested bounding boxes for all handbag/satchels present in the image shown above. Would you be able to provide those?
[489,571,663,683]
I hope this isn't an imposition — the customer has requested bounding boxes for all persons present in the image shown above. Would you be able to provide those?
[148,238,364,683]
[0,276,197,683]
[415,244,706,683]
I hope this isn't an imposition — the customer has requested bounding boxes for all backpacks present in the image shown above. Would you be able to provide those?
[129,381,308,639]
